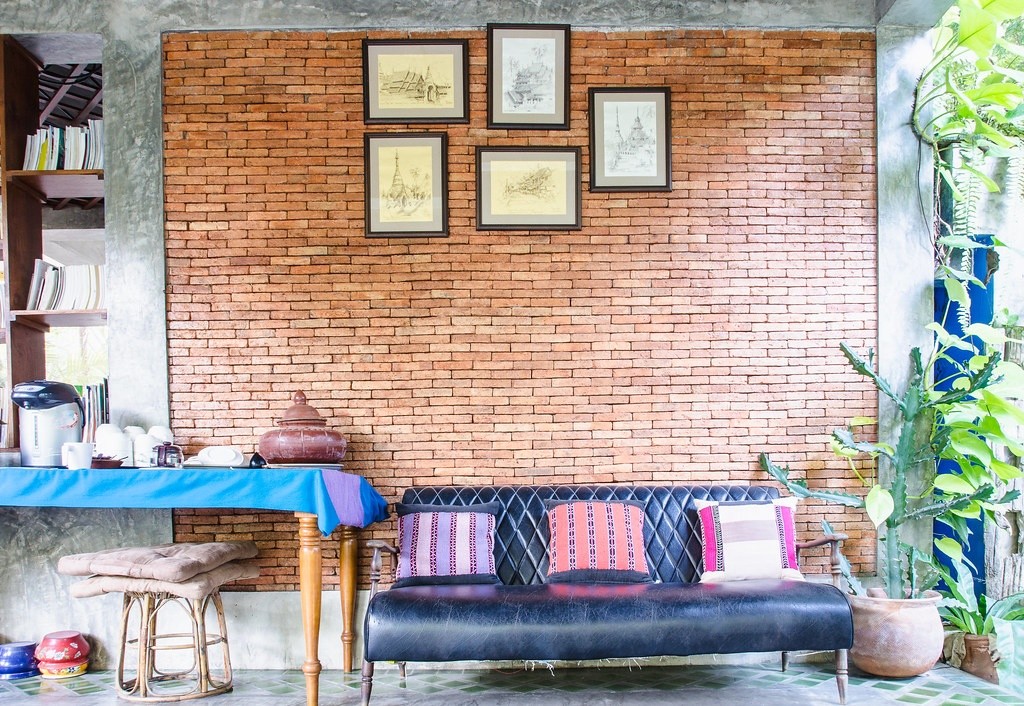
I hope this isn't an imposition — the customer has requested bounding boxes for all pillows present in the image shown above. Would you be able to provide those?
[389,501,503,591]
[545,498,656,586]
[67,560,260,599]
[694,496,806,583]
[56,539,258,584]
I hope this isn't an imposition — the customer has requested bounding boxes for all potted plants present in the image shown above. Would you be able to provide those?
[926,559,1024,685]
[756,343,1003,677]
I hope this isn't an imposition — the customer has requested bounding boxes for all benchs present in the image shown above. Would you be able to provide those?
[361,487,854,706]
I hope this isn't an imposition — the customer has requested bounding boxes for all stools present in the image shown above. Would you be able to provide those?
[115,592,233,702]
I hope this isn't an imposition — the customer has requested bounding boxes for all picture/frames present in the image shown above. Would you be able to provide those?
[475,145,582,230]
[486,22,571,131]
[589,87,673,192]
[361,39,471,125]
[363,131,450,239]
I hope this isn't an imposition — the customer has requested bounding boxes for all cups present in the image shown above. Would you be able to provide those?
[61,442,93,470]
[149,441,184,468]
[95,425,173,467]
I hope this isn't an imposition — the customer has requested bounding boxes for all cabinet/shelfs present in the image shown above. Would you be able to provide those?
[0,34,106,454]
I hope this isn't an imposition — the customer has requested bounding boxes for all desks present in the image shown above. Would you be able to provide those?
[0,466,387,706]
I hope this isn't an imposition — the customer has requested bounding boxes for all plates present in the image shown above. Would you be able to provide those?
[199,446,243,466]
[92,460,123,468]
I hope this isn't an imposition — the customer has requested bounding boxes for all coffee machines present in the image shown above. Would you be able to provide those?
[11,380,86,468]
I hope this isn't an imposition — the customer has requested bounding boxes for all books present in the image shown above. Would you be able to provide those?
[25,119,103,169]
[77,379,109,445]
[26,259,104,311]
